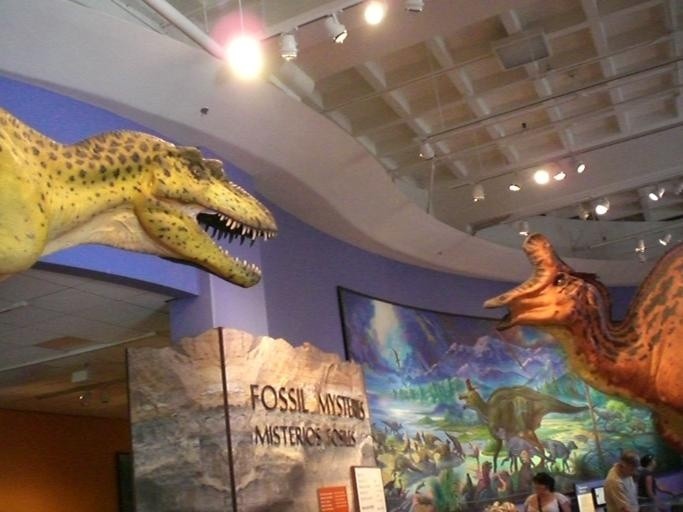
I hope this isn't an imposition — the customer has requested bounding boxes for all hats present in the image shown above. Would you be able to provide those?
[640,454,654,468]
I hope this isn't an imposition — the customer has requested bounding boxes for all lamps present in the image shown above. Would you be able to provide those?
[418,140,671,264]
[276,13,347,61]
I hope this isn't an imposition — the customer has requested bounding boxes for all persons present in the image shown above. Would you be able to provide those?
[640,454,680,512]
[524,472,571,512]
[604,452,643,512]
